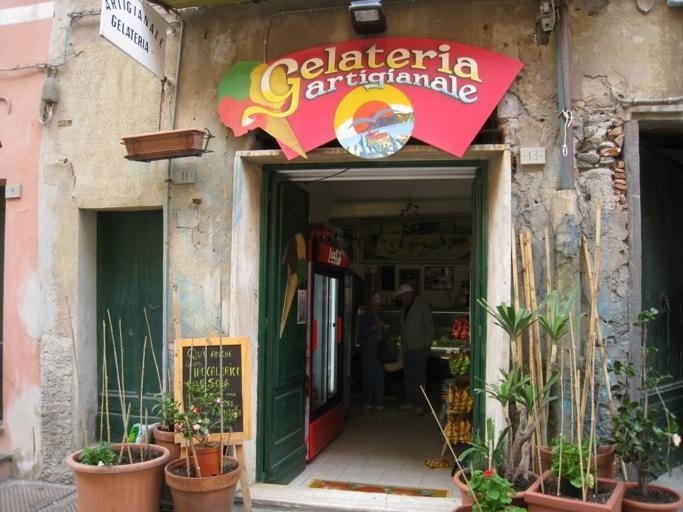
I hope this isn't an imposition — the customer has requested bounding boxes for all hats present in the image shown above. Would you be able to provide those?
[394,284,414,297]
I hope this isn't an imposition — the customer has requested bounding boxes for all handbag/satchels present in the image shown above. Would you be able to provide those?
[378,338,398,364]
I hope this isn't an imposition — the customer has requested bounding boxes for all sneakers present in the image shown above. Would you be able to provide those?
[415,406,425,415]
[364,404,387,411]
[401,403,415,410]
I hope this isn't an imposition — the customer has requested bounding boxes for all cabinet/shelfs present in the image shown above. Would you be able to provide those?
[351,304,470,401]
[436,334,472,462]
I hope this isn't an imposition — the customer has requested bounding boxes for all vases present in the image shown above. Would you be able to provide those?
[66,379,243,512]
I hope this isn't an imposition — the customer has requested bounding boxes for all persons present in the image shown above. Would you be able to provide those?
[393,283,435,416]
[358,290,389,411]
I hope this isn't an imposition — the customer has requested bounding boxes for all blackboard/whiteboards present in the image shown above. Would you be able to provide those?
[174,336,252,443]
